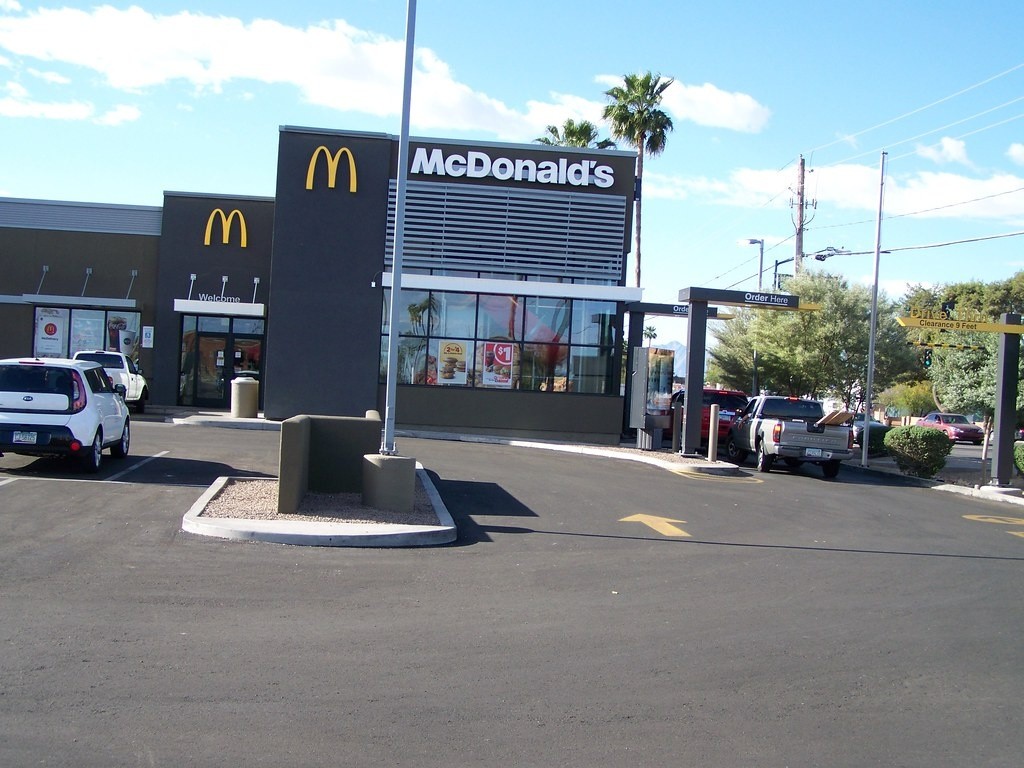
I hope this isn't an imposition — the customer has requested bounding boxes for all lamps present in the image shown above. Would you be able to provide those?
[371,271,381,288]
[220,275,229,301]
[81,267,93,297]
[125,269,137,299]
[186,274,197,299]
[36,265,49,293]
[251,277,260,303]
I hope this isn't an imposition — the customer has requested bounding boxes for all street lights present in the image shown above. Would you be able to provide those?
[736,239,764,398]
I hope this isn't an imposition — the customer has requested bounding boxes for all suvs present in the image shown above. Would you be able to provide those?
[73,350,149,415]
[0,358,130,471]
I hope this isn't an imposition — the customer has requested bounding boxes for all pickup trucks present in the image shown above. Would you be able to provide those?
[725,395,854,479]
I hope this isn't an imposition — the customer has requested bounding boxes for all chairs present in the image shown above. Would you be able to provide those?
[56,376,71,388]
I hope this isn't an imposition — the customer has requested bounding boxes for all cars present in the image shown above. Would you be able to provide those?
[1014,427,1024,441]
[917,413,985,445]
[661,389,749,447]
[840,413,885,443]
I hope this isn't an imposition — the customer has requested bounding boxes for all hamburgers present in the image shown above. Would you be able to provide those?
[439,357,465,378]
[493,365,510,378]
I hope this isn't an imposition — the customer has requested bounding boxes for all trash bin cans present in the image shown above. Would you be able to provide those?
[230,377,259,418]
